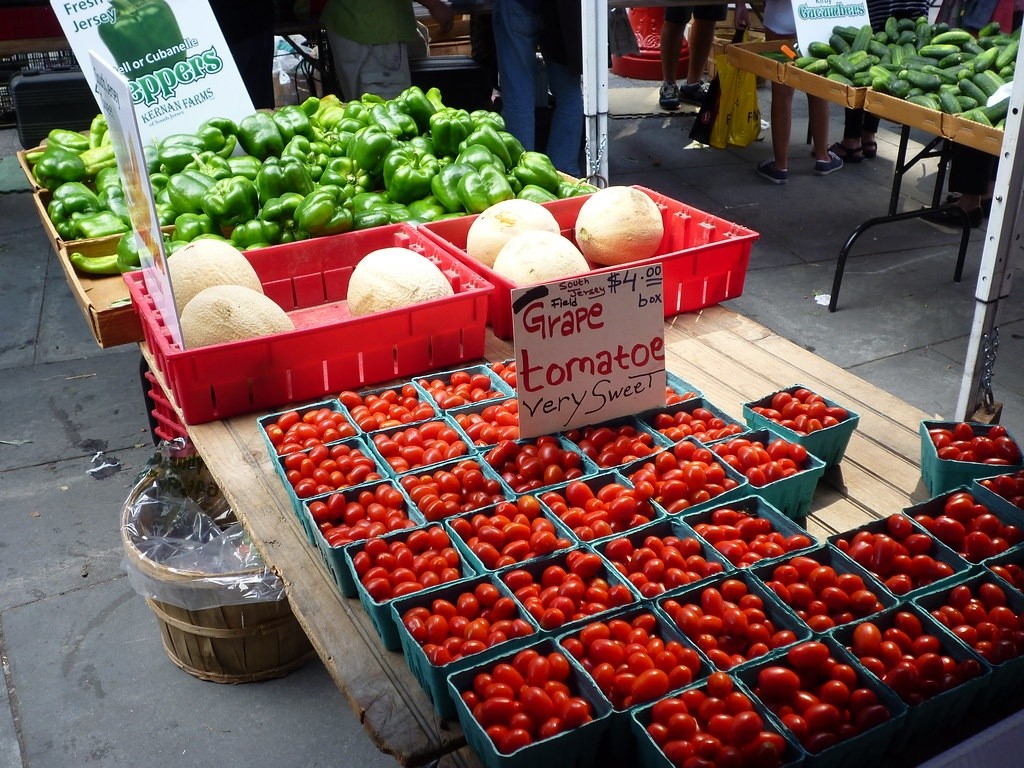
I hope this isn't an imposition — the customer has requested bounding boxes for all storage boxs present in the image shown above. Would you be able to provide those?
[255,357,1024,768]
[727,38,1004,157]
[142,370,191,442]
[417,184,765,342]
[17,145,145,350]
[120,183,495,426]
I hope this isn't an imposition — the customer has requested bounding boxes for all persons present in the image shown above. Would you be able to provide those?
[209,0,1024,231]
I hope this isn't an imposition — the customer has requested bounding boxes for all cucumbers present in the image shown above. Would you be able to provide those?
[794,17,1023,131]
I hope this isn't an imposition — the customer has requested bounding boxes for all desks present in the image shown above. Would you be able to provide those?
[135,300,939,768]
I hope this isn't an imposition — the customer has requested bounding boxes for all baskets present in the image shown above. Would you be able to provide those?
[418,184,760,340]
[144,372,187,443]
[256,356,1024,768]
[122,222,497,425]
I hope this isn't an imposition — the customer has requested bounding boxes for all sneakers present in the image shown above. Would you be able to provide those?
[679,80,710,107]
[659,80,681,108]
[814,151,843,175]
[755,157,789,184]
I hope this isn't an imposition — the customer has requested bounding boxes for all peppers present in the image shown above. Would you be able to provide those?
[23,112,143,275]
[142,83,594,261]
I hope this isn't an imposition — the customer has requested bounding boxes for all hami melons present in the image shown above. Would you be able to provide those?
[166,239,296,352]
[466,186,663,289]
[347,247,455,317]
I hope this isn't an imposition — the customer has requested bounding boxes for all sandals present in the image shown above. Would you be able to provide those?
[864,139,877,156]
[812,143,864,162]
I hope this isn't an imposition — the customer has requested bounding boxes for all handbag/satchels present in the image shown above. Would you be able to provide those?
[689,24,764,148]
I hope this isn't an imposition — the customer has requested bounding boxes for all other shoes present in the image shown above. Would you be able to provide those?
[922,204,984,227]
[948,193,992,218]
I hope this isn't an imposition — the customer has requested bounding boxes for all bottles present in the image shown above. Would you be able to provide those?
[10,65,102,150]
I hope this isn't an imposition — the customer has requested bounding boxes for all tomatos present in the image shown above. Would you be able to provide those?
[265,359,1024,768]
